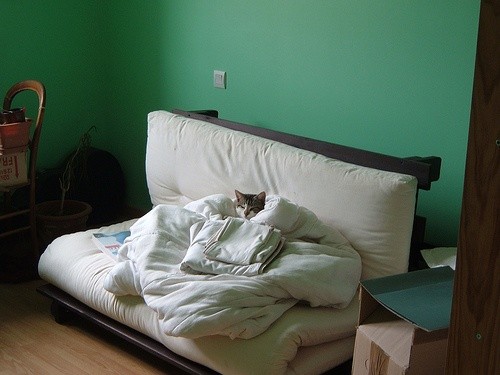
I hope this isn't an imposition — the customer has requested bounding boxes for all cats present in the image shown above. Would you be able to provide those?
[235,188,267,219]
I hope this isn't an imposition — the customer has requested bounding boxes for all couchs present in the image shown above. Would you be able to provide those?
[37,108,441,375]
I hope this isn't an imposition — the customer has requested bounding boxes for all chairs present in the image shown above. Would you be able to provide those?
[0,79,47,278]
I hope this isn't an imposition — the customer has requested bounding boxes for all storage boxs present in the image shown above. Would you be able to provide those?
[351,264,455,375]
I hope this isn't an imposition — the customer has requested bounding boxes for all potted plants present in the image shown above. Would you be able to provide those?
[38,125,97,257]
